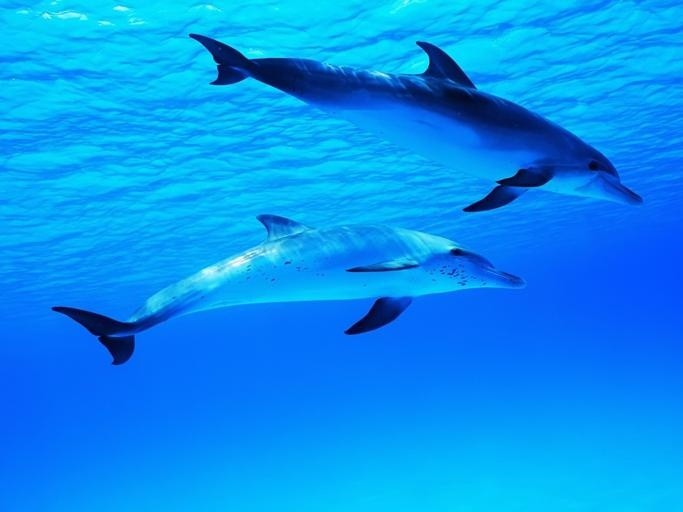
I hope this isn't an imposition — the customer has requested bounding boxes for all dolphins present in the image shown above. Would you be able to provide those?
[51,215,528,366]
[187,31,644,213]
